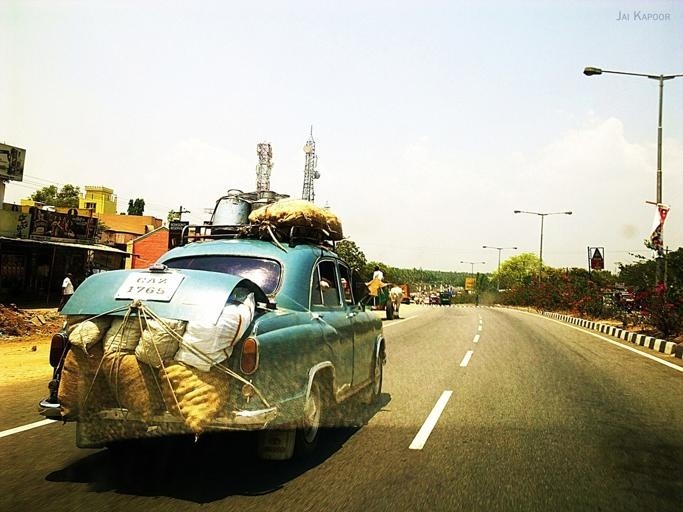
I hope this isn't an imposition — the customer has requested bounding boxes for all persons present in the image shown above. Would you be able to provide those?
[56,272,75,312]
[368,266,386,296]
[46,214,76,237]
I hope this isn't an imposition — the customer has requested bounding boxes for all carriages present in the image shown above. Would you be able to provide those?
[362,286,405,319]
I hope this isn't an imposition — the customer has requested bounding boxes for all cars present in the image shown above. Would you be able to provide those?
[37,240,386,459]
[430,292,451,305]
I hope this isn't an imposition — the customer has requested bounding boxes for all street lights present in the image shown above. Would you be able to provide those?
[584,66,682,288]
[483,246,517,290]
[514,211,572,288]
[461,261,485,278]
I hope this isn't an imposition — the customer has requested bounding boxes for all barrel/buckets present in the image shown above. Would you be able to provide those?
[211,197,250,237]
